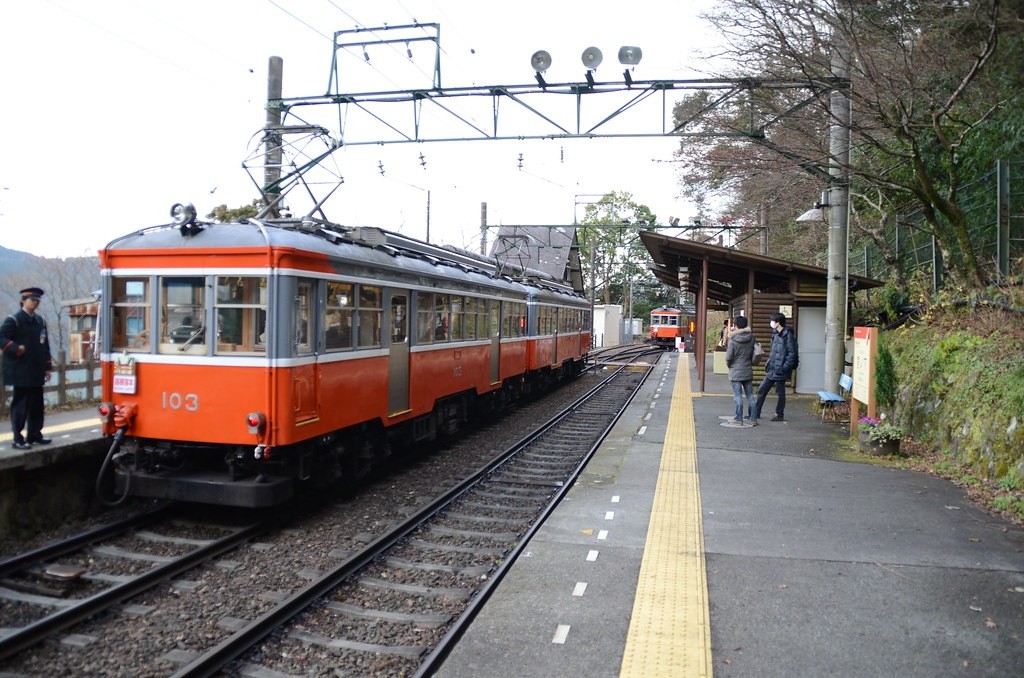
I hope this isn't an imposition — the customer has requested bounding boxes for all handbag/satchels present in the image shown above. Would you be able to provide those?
[752,342,765,365]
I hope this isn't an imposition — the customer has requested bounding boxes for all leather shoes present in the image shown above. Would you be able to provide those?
[28,438,51,444]
[13,440,31,449]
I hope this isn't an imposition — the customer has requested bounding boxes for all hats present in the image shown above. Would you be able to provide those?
[20,287,44,301]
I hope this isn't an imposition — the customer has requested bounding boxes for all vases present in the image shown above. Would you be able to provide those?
[859,431,879,453]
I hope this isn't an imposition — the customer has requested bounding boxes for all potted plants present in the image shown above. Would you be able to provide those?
[867,423,903,454]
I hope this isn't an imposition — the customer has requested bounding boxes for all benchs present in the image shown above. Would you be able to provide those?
[817,374,852,424]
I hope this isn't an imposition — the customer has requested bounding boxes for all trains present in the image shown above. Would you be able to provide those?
[650,305,696,349]
[99,202,593,523]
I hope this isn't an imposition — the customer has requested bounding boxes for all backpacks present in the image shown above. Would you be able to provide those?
[793,355,799,368]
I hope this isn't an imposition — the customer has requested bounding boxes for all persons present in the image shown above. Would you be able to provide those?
[0,286,53,450]
[213,282,483,361]
[744,312,800,421]
[725,316,764,426]
[720,320,727,347]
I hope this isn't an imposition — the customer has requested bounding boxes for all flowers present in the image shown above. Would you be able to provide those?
[858,416,877,431]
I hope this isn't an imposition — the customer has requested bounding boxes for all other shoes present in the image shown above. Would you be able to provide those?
[771,415,783,421]
[745,414,760,419]
[728,419,743,426]
[743,420,758,425]
